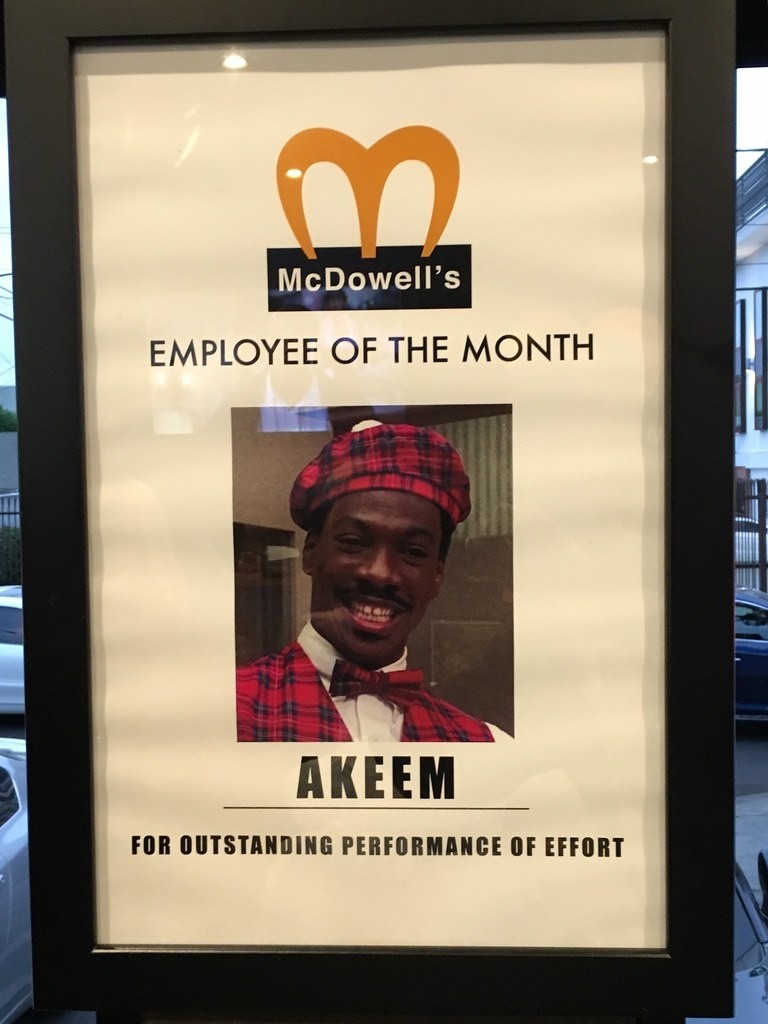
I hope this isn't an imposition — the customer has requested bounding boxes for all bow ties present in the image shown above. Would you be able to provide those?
[329,659,424,709]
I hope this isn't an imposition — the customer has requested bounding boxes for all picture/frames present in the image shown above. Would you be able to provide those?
[5,0,744,1024]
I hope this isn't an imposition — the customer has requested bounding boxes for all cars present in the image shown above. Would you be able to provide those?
[734,584,768,722]
[687,851,768,1024]
[0,584,25,716]
[735,516,768,568]
[0,744,31,1024]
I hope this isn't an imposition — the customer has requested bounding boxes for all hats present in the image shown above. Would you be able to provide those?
[289,425,471,531]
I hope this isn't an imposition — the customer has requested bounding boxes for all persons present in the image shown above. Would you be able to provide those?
[235,422,514,742]
